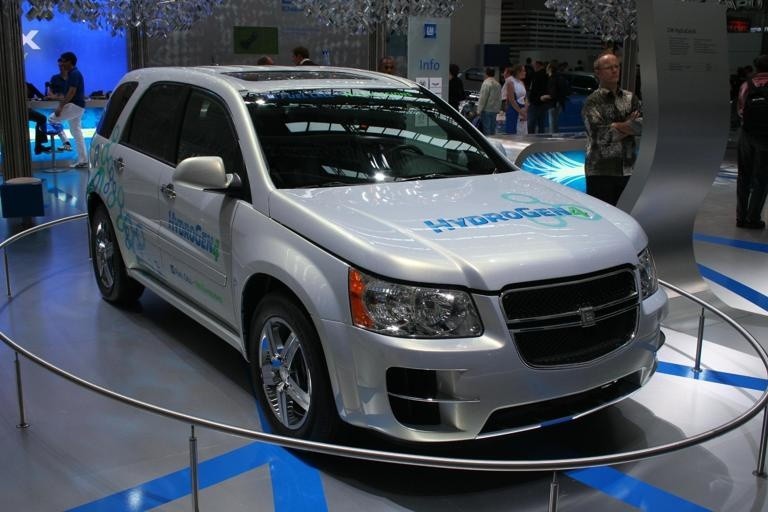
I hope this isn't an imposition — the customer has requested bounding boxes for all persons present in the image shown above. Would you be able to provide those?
[499,67,516,111]
[477,66,503,137]
[732,54,768,229]
[46,58,70,101]
[578,48,644,207]
[448,63,467,109]
[46,51,91,170]
[503,64,530,135]
[728,64,760,131]
[25,82,51,154]
[377,56,395,74]
[524,57,574,134]
[290,46,319,66]
[256,56,274,66]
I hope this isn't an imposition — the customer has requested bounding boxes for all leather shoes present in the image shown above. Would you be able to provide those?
[34,144,89,168]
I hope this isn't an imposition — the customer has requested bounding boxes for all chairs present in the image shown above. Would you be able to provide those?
[200,102,283,192]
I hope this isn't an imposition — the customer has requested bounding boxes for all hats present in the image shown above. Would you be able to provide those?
[753,55,767,72]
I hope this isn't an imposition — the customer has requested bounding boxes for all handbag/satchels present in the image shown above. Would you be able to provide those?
[516,116,530,138]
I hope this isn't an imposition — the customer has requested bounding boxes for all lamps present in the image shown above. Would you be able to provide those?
[544,0,639,44]
[5,1,229,39]
[298,0,457,37]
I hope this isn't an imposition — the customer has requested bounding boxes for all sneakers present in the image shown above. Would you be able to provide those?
[736,219,766,230]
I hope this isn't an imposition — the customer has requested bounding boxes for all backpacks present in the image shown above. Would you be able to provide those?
[742,76,768,136]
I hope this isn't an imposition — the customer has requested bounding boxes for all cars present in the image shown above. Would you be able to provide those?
[86,65,668,454]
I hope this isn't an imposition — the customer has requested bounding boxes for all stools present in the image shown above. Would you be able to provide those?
[1,176,47,237]
[38,123,69,174]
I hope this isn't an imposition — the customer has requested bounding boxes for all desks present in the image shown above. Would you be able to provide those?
[27,99,108,109]
